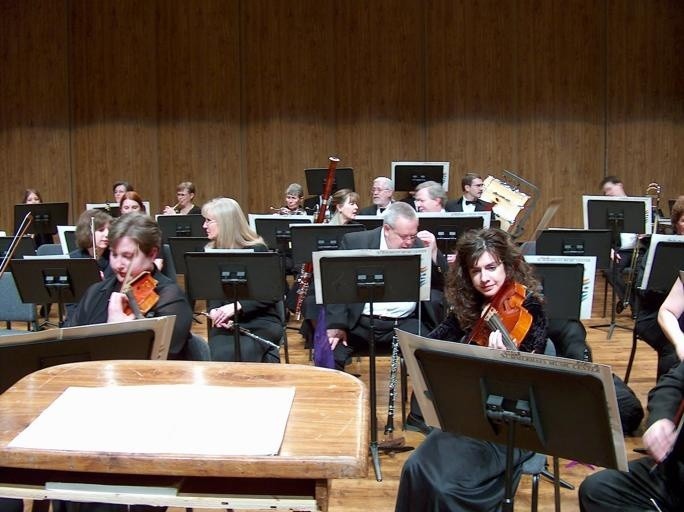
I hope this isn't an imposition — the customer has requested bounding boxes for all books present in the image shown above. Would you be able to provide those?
[6,384,296,457]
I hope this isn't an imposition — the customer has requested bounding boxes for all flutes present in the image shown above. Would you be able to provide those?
[173,200,182,210]
[90,217,96,259]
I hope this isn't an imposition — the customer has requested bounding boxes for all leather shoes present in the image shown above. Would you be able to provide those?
[405,414,432,435]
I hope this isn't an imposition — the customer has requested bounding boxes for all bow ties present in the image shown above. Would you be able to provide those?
[466,200,478,205]
[380,207,386,213]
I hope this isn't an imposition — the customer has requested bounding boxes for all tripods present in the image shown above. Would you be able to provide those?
[367,302,416,481]
[588,234,636,340]
[31,305,60,333]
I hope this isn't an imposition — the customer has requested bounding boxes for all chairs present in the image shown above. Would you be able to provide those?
[601,267,631,318]
[273,300,289,363]
[0,272,37,336]
[520,338,558,512]
[519,242,537,255]
[182,334,211,361]
[622,330,661,385]
[161,244,177,284]
[36,244,63,256]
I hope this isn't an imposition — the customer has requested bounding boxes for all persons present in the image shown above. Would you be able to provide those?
[598,175,655,318]
[69,182,201,275]
[636,197,684,381]
[68,212,193,360]
[579,363,683,512]
[395,228,551,512]
[326,202,449,432]
[278,174,495,349]
[202,198,283,363]
[22,189,57,315]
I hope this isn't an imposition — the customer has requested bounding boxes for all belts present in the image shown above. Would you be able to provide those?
[365,314,403,321]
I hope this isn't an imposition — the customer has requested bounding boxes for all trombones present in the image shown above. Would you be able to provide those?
[623,182,661,309]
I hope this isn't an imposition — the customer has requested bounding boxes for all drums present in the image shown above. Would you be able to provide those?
[479,175,530,232]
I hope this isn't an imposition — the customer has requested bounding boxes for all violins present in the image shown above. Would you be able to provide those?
[468,279,533,351]
[121,270,160,320]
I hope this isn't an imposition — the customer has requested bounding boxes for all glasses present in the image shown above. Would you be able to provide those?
[387,223,418,240]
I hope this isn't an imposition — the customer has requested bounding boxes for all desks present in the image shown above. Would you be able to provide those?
[0,359,371,512]
